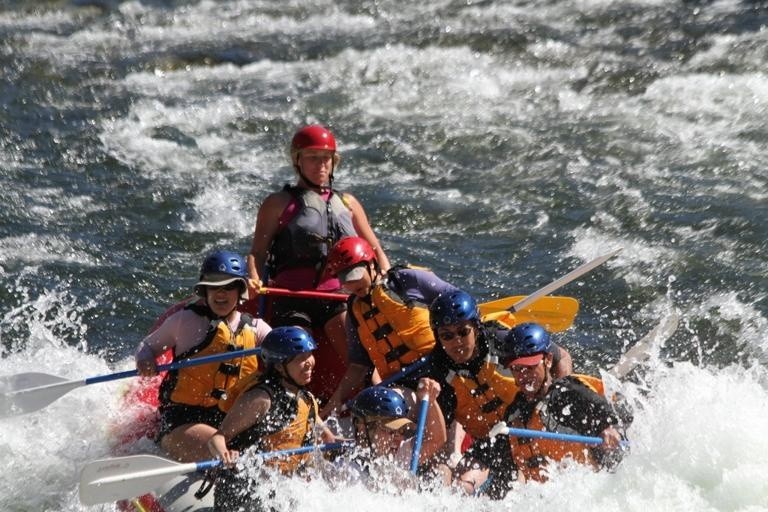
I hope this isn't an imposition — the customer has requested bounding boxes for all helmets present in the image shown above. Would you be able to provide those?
[292,124,336,153]
[201,250,249,279]
[351,385,409,419]
[327,236,378,279]
[429,289,480,330]
[261,325,319,364]
[502,322,551,360]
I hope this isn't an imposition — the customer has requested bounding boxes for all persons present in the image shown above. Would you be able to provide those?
[334,377,448,488]
[206,326,336,512]
[424,289,574,442]
[247,126,392,369]
[317,236,459,418]
[487,321,625,504]
[137,250,273,467]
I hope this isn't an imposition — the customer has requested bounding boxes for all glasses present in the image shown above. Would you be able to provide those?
[205,285,238,290]
[437,328,473,341]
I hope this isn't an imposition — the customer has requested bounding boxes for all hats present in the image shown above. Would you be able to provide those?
[194,273,248,298]
[368,416,414,430]
[504,352,544,369]
[337,261,367,285]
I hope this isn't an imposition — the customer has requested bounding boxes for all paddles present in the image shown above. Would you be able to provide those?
[0,346,263,423]
[332,247,623,412]
[604,312,680,383]
[78,442,348,506]
[257,281,577,334]
